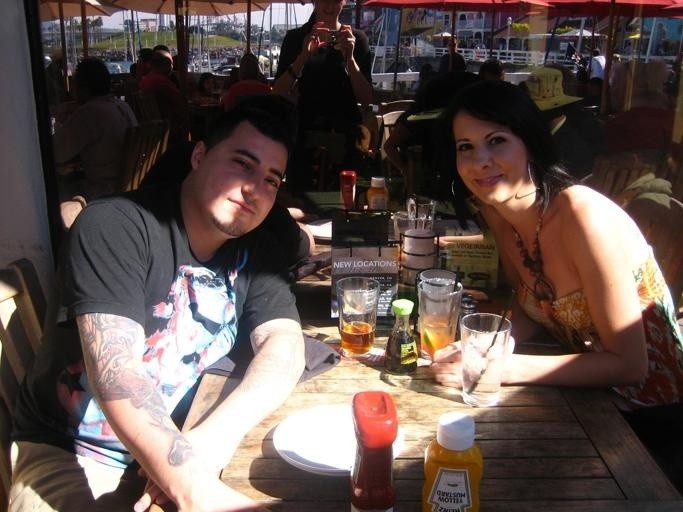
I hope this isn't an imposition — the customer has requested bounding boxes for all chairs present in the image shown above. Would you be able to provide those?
[378,105,428,199]
[0,258,44,512]
[623,195,683,324]
[121,118,169,194]
[592,151,661,204]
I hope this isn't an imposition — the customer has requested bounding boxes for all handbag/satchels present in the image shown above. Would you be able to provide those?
[332,239,399,325]
[332,208,390,248]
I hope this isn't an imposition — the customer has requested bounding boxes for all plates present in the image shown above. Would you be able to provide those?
[272,400,406,478]
[306,219,332,240]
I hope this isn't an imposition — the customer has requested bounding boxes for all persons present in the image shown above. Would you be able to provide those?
[273,0,374,191]
[378,76,447,175]
[135,47,154,95]
[5,104,306,512]
[145,52,187,117]
[478,58,505,82]
[439,81,683,497]
[129,59,138,79]
[439,54,469,100]
[586,50,606,86]
[42,52,66,102]
[152,43,170,54]
[413,63,434,90]
[228,54,270,88]
[39,59,139,204]
[191,68,217,99]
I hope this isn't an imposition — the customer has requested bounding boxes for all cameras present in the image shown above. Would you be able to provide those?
[325,30,345,43]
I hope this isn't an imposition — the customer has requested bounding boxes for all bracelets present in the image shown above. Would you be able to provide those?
[288,64,303,80]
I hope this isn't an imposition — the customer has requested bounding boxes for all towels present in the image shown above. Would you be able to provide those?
[200,330,341,384]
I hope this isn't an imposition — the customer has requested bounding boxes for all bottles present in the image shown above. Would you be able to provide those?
[385,299,417,375]
[350,392,398,512]
[422,413,482,512]
[340,171,356,210]
[367,177,388,210]
[476,45,481,58]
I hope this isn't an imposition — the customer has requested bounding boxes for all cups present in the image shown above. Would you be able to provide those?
[393,210,426,265]
[406,195,436,236]
[336,277,380,361]
[417,277,464,362]
[460,312,512,409]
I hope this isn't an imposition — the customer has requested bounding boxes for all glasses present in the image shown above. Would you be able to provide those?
[448,43,457,47]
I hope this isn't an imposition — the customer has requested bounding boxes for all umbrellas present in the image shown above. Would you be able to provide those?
[364,0,540,55]
[433,32,455,46]
[107,0,271,60]
[38,0,127,57]
[495,12,599,55]
[557,27,601,56]
[548,0,683,69]
